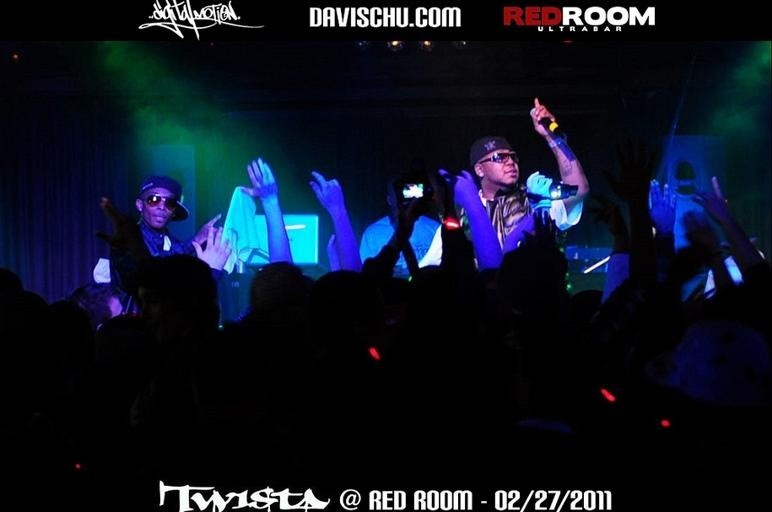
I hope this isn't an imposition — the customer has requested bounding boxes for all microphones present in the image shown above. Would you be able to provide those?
[530,108,564,139]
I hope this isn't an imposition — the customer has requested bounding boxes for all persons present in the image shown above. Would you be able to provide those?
[0,97,772,511]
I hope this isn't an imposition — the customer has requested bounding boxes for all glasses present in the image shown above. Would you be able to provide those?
[479,152,520,163]
[147,195,177,210]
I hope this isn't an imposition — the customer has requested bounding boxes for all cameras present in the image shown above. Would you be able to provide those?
[400,182,433,201]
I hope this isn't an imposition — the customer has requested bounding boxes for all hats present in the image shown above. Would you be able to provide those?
[470,135,515,166]
[137,175,189,222]
